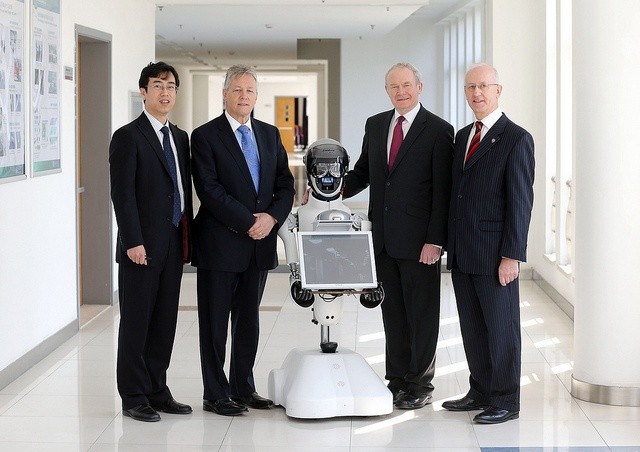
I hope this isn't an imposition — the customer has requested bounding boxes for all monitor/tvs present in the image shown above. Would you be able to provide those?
[298,231,378,289]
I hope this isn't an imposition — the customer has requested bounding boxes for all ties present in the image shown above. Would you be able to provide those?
[465,121,483,167]
[237,125,260,195]
[388,116,405,175]
[160,126,181,227]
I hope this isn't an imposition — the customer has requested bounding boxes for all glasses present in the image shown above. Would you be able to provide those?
[145,83,179,92]
[465,82,499,90]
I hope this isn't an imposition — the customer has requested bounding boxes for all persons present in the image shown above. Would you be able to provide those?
[109,62,193,422]
[302,62,454,410]
[190,65,297,414]
[447,65,534,423]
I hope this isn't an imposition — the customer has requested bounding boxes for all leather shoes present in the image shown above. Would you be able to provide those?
[151,398,192,415]
[231,393,273,409]
[442,397,490,411]
[122,403,161,422]
[473,407,519,424]
[388,382,433,409]
[203,398,249,416]
